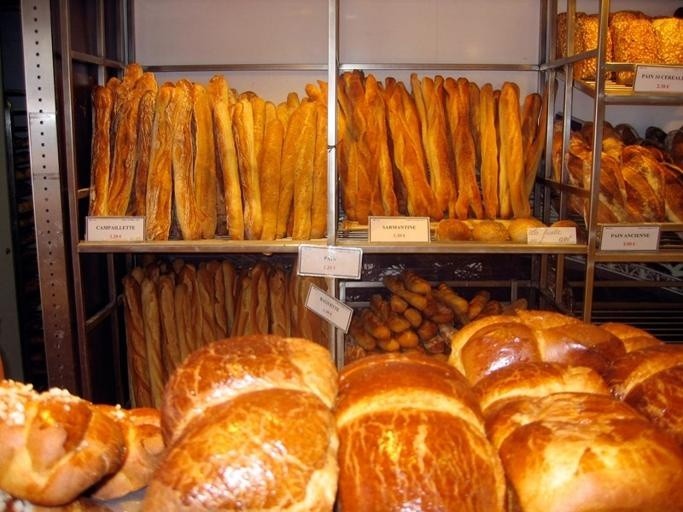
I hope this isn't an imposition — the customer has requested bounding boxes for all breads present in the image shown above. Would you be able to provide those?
[241,90,263,158]
[336,69,357,222]
[0,378,162,512]
[611,9,666,85]
[230,95,262,241]
[365,75,397,216]
[558,11,587,58]
[292,103,317,242]
[469,82,480,136]
[123,63,143,90]
[286,92,300,115]
[573,15,612,81]
[444,76,484,219]
[345,74,369,225]
[409,72,427,143]
[135,72,157,96]
[500,92,542,217]
[508,218,548,243]
[344,272,526,362]
[160,334,339,445]
[106,76,132,98]
[172,78,198,239]
[311,105,330,243]
[455,77,477,218]
[526,79,559,198]
[132,90,156,217]
[498,82,531,218]
[602,343,683,447]
[316,78,327,91]
[206,74,244,241]
[420,77,457,200]
[278,103,287,126]
[600,321,662,354]
[551,118,683,240]
[144,388,339,512]
[435,218,472,242]
[275,109,302,240]
[479,83,500,221]
[145,86,173,242]
[161,261,327,349]
[334,351,507,512]
[110,88,140,216]
[121,268,160,407]
[263,101,276,130]
[473,221,509,244]
[88,85,111,217]
[261,118,285,241]
[385,76,442,220]
[304,83,321,102]
[472,361,683,511]
[653,15,683,66]
[426,75,451,211]
[398,80,423,216]
[447,309,624,386]
[190,83,216,237]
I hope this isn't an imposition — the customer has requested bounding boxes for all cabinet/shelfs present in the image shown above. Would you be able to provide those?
[60,0,683,401]
